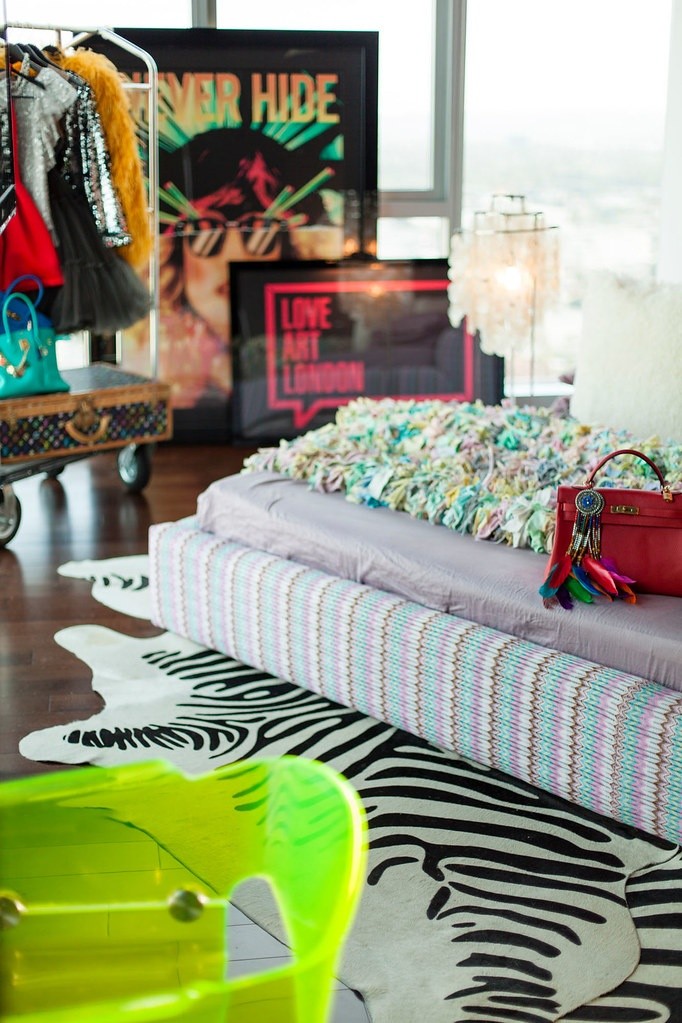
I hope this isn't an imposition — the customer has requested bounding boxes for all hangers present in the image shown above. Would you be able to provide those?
[9,23,41,72]
[11,68,47,91]
[42,26,65,61]
[16,20,81,86]
[27,23,63,70]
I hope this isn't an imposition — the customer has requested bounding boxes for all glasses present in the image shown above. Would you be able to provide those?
[174,213,288,258]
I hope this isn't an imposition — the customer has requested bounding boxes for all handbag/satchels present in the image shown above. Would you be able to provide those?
[539,446,680,610]
[1,272,54,333]
[1,291,71,399]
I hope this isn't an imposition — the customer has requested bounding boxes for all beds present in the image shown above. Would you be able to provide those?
[147,470,682,849]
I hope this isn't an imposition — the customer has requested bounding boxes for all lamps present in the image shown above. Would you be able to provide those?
[446,190,564,405]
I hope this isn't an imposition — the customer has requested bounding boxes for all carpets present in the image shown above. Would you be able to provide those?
[17,552,682,1023]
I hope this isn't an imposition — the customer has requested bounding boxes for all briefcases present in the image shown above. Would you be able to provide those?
[0,361,173,462]
